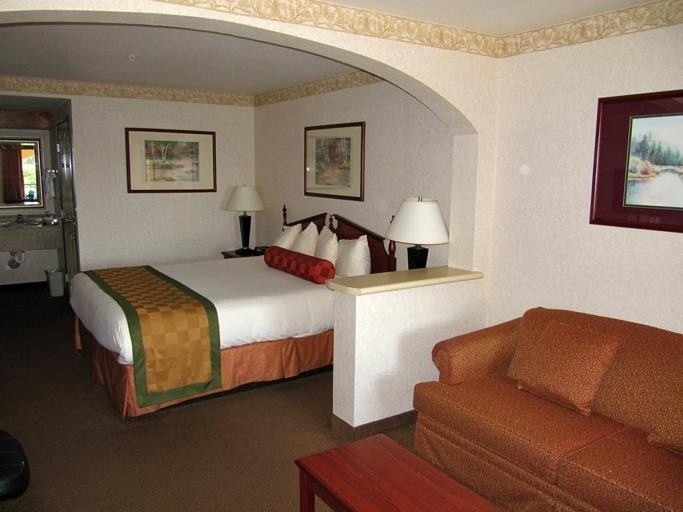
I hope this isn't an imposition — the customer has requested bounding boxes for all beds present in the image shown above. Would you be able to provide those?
[68,204,396,418]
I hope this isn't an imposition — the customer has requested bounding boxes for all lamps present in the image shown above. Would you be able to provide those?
[385,198,449,269]
[226,183,265,255]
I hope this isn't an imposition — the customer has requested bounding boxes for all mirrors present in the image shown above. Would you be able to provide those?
[0,128,55,216]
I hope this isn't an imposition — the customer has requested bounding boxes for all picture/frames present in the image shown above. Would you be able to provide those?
[589,89,683,233]
[304,121,366,201]
[124,127,217,193]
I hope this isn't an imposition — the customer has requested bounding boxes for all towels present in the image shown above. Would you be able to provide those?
[44,168,56,199]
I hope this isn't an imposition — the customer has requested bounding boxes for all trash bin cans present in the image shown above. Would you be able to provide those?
[45,268,65,297]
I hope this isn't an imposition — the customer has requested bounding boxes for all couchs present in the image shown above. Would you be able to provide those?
[413,307,683,512]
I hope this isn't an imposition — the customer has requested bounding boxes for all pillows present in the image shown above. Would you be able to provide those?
[517,319,621,416]
[646,391,683,453]
[264,221,372,285]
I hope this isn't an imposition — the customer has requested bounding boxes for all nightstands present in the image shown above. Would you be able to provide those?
[221,249,261,258]
